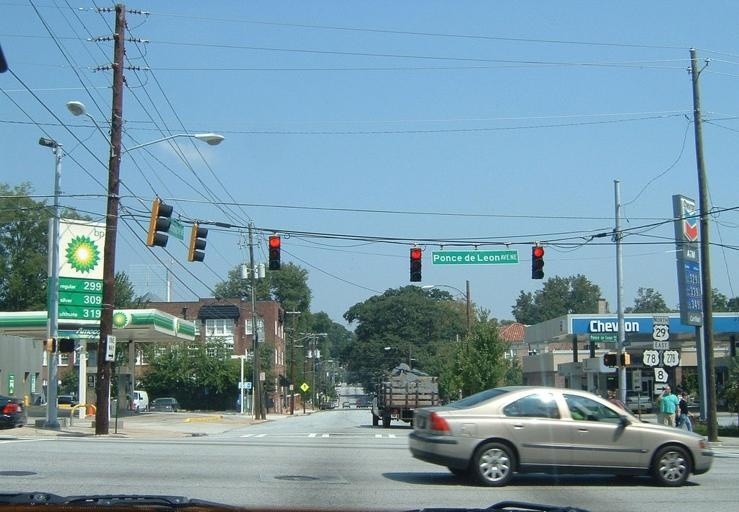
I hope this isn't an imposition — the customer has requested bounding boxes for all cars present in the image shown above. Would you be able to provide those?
[408,383,716,488]
[59,395,78,404]
[0,393,30,432]
[355,398,373,409]
[624,388,653,413]
[150,397,181,413]
[341,401,350,408]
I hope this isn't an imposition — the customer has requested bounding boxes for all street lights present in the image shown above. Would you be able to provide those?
[421,280,473,340]
[309,330,346,411]
[381,346,412,370]
[62,96,231,440]
[35,136,63,432]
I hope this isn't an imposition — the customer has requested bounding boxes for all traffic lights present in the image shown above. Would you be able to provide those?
[406,247,424,282]
[46,338,57,354]
[620,354,629,367]
[58,336,74,356]
[529,246,546,280]
[602,352,619,368]
[145,200,173,248]
[186,224,209,263]
[267,234,283,270]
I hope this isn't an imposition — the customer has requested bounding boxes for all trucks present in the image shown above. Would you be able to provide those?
[371,372,441,428]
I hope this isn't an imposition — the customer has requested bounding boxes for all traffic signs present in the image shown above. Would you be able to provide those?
[164,217,185,240]
[430,249,521,266]
[105,333,118,363]
[588,333,619,343]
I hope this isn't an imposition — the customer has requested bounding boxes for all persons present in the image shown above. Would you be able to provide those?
[658,386,680,429]
[565,399,592,420]
[677,393,694,433]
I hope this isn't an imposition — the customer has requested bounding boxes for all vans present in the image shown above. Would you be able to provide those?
[132,390,150,412]
